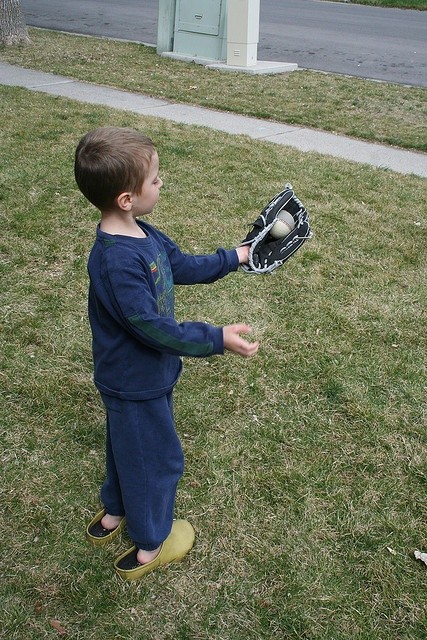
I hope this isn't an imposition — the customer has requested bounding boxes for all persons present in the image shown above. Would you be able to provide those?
[74,125,260,580]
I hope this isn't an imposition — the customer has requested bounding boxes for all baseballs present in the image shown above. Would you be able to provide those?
[270,209,295,240]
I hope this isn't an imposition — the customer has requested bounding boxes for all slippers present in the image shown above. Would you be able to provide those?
[87,509,127,545]
[113,519,194,579]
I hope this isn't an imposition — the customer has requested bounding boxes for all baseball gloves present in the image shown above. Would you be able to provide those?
[238,187,312,276]
